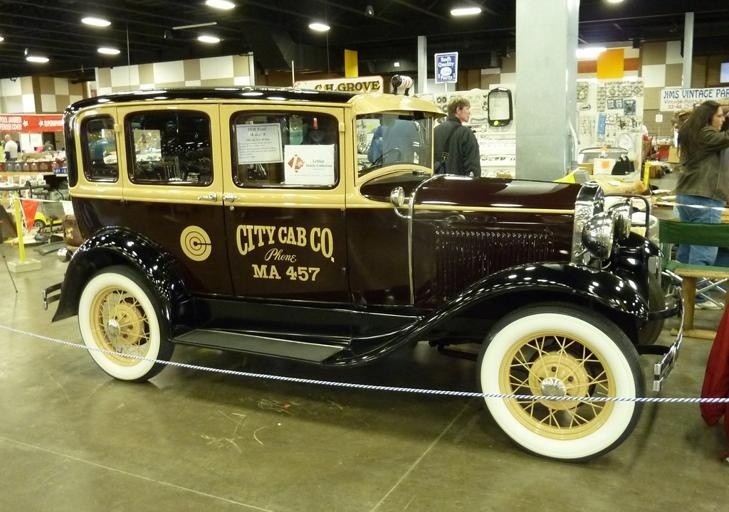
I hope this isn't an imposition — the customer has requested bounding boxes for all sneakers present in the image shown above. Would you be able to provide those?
[694,301,724,310]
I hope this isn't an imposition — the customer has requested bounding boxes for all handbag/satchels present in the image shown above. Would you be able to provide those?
[368,136,383,163]
[435,160,444,174]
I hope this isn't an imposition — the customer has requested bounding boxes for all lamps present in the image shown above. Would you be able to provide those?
[308,18,331,33]
[196,30,222,44]
[80,14,121,56]
[24,50,50,64]
[448,0,483,17]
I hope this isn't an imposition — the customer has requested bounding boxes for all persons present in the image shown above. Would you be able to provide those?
[434,97,481,177]
[675,100,729,309]
[5,135,18,161]
[368,120,427,165]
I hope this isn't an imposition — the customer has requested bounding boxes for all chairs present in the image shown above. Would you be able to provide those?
[300,129,339,185]
[655,218,728,340]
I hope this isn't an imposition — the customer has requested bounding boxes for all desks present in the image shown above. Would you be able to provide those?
[1,196,62,246]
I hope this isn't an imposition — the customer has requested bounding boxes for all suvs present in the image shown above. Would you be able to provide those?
[42,86,686,463]
